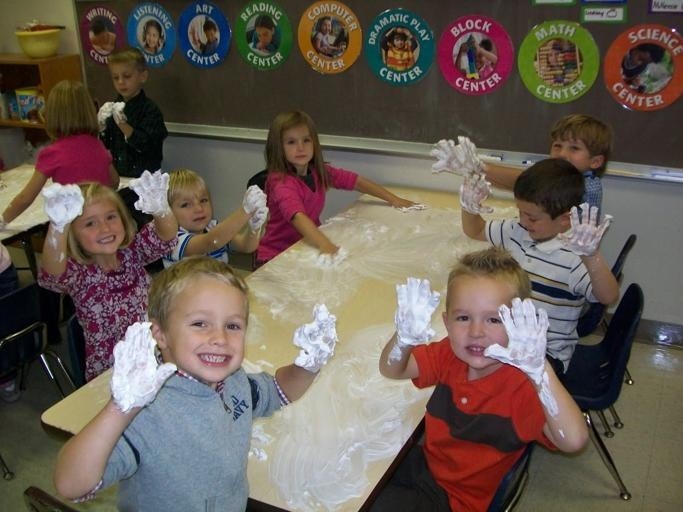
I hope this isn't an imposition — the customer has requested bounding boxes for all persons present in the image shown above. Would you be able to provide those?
[0,77,120,226]
[312,17,348,55]
[50,255,339,511]
[96,47,167,178]
[189,20,217,57]
[361,246,593,512]
[455,39,498,79]
[457,151,619,384]
[252,16,281,55]
[34,168,181,381]
[142,21,165,55]
[158,169,271,265]
[1,241,22,405]
[251,109,430,268]
[543,39,569,86]
[88,16,117,54]
[621,43,670,85]
[428,111,617,320]
[386,32,416,72]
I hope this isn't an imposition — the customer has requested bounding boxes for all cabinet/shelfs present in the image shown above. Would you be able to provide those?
[1,54,84,132]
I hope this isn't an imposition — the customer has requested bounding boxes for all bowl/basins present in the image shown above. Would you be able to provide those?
[13,30,62,60]
